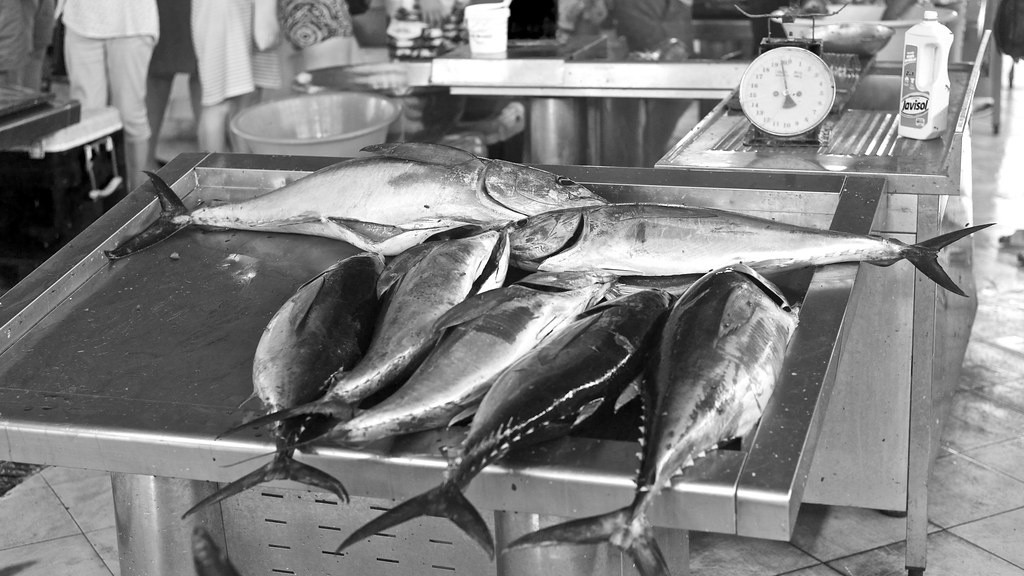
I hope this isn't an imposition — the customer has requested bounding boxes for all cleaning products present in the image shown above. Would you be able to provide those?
[897,8,954,140]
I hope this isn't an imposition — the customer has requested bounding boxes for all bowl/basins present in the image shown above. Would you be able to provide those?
[802,22,895,57]
[230,92,401,158]
[289,63,464,143]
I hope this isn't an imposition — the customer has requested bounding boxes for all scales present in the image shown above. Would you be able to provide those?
[734,3,849,147]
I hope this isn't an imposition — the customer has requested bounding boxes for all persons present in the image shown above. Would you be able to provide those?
[190,1,470,153]
[992,1,1024,266]
[508,0,694,167]
[0,0,161,195]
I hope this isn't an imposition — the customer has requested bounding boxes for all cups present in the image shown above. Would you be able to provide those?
[463,4,510,53]
[822,55,861,93]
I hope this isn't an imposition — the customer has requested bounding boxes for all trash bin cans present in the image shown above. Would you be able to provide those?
[461,96,589,165]
[0,105,129,261]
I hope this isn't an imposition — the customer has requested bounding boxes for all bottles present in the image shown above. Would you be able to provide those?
[898,10,953,140]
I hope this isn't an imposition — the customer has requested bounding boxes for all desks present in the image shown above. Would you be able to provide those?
[5,0,1000,574]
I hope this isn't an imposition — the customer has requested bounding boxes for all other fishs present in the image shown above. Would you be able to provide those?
[104,142,997,576]
